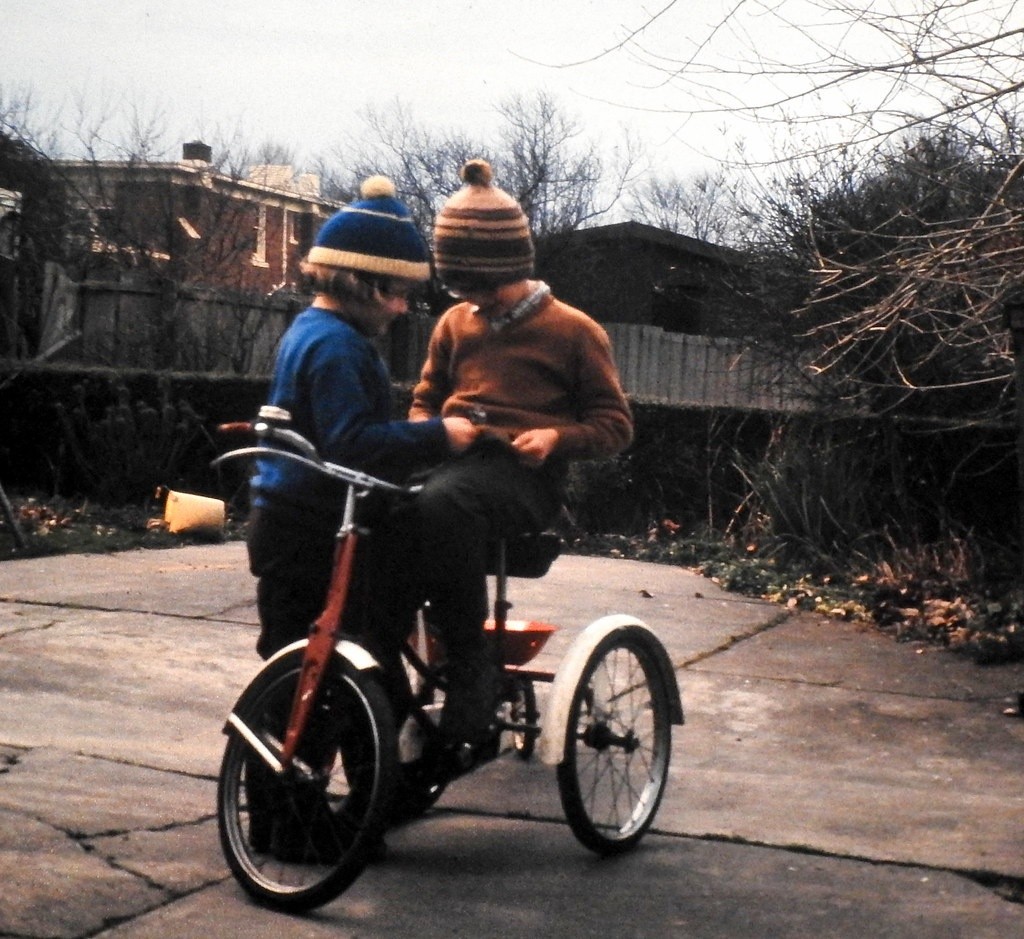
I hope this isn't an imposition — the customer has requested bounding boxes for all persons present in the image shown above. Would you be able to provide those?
[408,159,634,776]
[244,174,477,862]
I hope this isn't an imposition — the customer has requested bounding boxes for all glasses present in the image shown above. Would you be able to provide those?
[441,278,509,305]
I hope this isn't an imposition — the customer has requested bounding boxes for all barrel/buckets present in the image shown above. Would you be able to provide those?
[164,490,225,538]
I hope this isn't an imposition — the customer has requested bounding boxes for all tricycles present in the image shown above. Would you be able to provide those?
[207,404,683,914]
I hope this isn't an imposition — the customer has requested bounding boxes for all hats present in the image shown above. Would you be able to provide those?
[434,160,536,282]
[308,176,431,280]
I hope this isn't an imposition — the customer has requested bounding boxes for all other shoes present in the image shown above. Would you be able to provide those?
[438,658,503,747]
[249,813,274,851]
[273,809,387,866]
[354,761,437,829]
